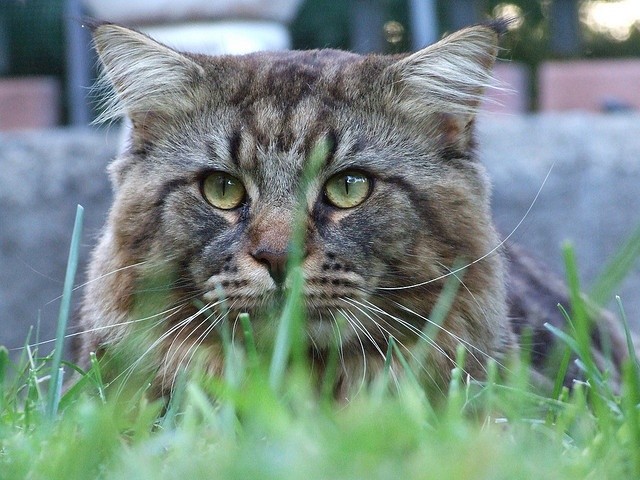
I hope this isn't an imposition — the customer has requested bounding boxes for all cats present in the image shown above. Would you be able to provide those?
[74,14,635,409]
